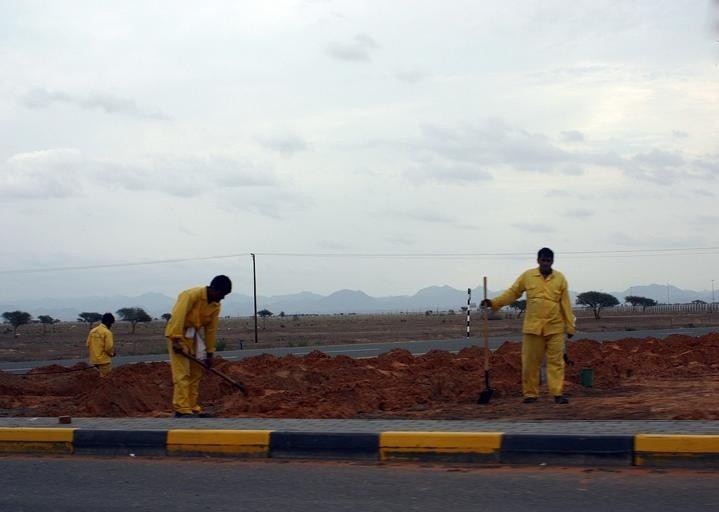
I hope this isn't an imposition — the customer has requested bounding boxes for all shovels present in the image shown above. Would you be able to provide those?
[476,276,496,404]
[182,351,265,396]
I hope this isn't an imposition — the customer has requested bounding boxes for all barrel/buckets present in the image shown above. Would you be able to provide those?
[580,368,594,386]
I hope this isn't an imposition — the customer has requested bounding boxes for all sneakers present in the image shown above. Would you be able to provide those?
[521,395,569,404]
[174,411,216,418]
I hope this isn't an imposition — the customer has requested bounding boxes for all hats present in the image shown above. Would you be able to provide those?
[101,313,114,324]
[211,274,231,293]
[537,247,553,258]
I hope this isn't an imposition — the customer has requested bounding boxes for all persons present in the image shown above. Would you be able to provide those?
[478,246,578,405]
[85,311,116,377]
[165,274,235,417]
